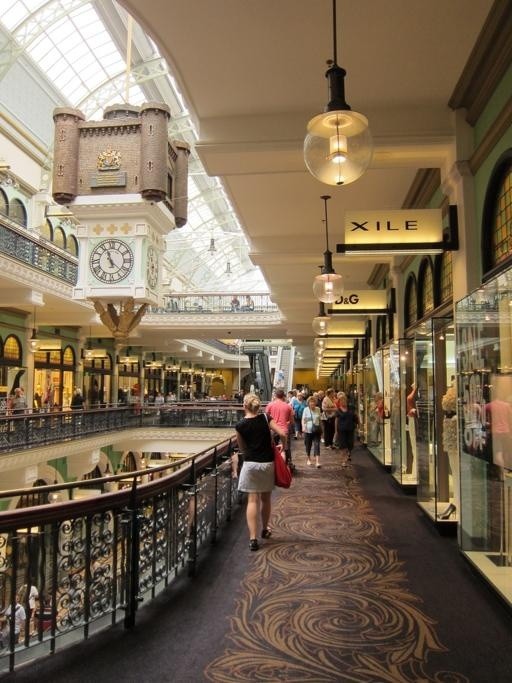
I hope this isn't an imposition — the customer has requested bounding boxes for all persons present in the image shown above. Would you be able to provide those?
[229,295,254,311]
[406,378,512,520]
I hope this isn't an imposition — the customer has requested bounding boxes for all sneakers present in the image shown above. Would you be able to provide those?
[248,538,260,552]
[319,437,339,451]
[306,459,323,470]
[340,455,353,469]
[261,526,272,539]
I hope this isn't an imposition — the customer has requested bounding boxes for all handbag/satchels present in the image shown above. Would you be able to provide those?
[311,424,323,435]
[272,436,294,489]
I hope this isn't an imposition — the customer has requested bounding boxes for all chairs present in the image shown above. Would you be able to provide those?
[162,299,255,312]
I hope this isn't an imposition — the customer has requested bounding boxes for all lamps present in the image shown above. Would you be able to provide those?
[150,352,223,380]
[307,197,348,305]
[122,345,133,367]
[310,298,326,383]
[206,237,217,256]
[223,260,234,276]
[290,0,375,192]
[25,306,42,353]
[81,323,97,361]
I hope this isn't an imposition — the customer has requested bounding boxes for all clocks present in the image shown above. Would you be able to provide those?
[144,243,162,290]
[87,236,134,285]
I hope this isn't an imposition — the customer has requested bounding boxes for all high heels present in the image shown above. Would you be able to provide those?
[438,504,457,520]
